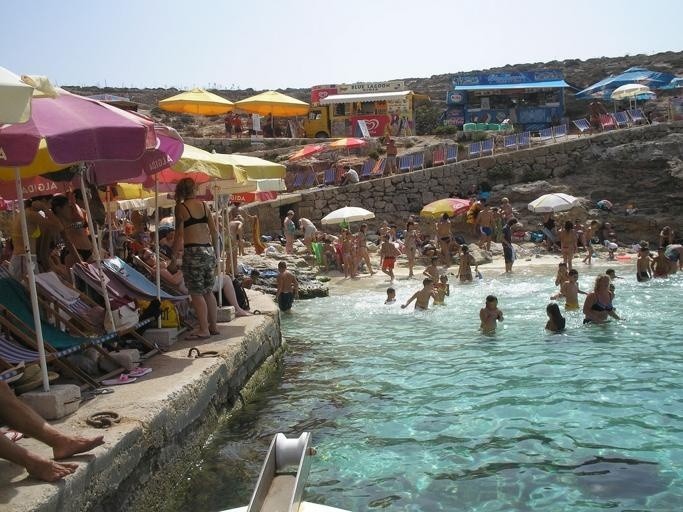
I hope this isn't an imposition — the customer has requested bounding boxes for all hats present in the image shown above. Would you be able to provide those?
[14,362,59,394]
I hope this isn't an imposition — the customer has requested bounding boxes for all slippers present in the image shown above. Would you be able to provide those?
[102,373,137,385]
[184,333,211,341]
[208,327,221,335]
[127,367,153,377]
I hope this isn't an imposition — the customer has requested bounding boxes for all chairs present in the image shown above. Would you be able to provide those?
[572,118,593,138]
[291,170,316,188]
[0,230,201,386]
[309,240,338,271]
[463,122,531,159]
[397,151,426,173]
[431,144,459,166]
[324,168,346,185]
[539,124,567,143]
[597,109,649,131]
[416,240,444,266]
[540,226,561,252]
[361,157,387,179]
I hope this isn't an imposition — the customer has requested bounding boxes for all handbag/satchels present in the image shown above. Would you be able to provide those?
[137,298,179,327]
[214,278,249,310]
[103,295,139,334]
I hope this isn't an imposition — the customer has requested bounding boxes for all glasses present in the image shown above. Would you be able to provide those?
[143,252,154,260]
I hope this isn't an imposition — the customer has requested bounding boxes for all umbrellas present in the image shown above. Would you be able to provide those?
[575,68,683,112]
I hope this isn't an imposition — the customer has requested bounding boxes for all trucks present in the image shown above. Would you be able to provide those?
[439,78,582,136]
[304,90,432,141]
